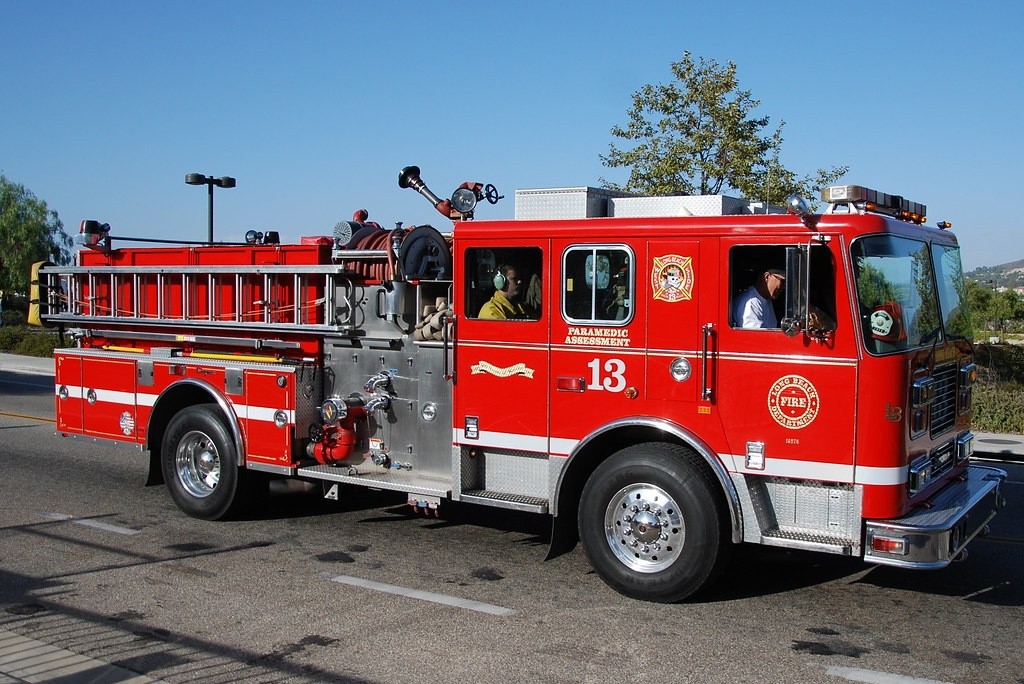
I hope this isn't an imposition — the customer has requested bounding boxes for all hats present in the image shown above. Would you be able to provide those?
[750,258,786,273]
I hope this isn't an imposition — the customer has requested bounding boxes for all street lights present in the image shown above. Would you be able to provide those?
[185,172,238,245]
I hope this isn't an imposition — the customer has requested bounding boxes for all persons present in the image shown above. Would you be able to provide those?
[730,259,827,330]
[478,263,532,320]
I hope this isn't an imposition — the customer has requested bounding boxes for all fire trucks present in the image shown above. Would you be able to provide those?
[28,164,1009,605]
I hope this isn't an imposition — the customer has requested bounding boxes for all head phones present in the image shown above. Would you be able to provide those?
[493,265,507,289]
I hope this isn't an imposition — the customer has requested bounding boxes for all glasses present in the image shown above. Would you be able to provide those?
[771,274,786,283]
